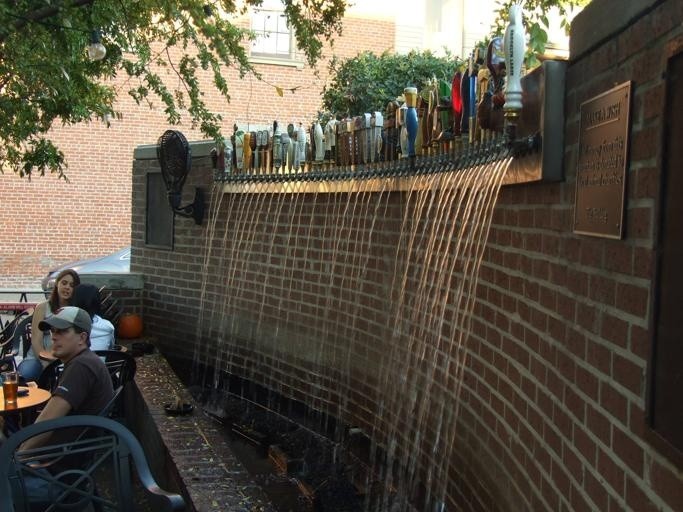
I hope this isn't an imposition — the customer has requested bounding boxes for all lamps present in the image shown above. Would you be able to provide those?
[156,129,204,223]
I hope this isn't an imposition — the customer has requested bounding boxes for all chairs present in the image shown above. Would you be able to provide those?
[0,305,186,512]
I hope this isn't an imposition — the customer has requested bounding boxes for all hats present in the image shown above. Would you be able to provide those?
[38,306,92,333]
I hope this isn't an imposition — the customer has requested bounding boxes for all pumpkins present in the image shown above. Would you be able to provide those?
[117,312,144,339]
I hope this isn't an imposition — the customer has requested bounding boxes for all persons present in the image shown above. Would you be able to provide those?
[14,268,82,385]
[56,282,117,373]
[1,305,113,511]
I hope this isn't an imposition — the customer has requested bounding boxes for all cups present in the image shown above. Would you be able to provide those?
[3,372,19,404]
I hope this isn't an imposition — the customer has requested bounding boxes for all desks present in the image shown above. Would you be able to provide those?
[0,288,52,319]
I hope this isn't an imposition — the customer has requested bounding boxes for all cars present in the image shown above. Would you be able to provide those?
[41,247,131,300]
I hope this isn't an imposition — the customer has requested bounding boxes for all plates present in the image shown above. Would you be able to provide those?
[163,404,193,416]
